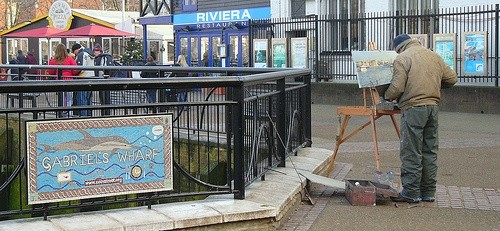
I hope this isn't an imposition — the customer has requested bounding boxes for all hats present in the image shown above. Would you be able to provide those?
[72,44,81,51]
[393,34,412,53]
[93,44,102,51]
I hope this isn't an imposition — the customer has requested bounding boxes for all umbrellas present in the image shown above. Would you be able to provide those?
[47,21,140,50]
[4,22,92,65]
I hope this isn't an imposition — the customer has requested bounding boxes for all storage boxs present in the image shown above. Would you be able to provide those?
[345,179,399,206]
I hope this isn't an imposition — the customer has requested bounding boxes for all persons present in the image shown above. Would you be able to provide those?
[384,33,459,204]
[47,43,80,117]
[71,44,95,116]
[93,44,114,115]
[140,56,159,111]
[67,49,75,60]
[202,48,220,76]
[167,55,191,115]
[6,49,39,80]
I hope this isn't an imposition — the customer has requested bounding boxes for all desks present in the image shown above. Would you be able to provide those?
[326,107,400,176]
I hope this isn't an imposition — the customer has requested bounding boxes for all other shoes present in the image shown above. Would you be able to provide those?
[390,195,419,203]
[421,199,434,202]
